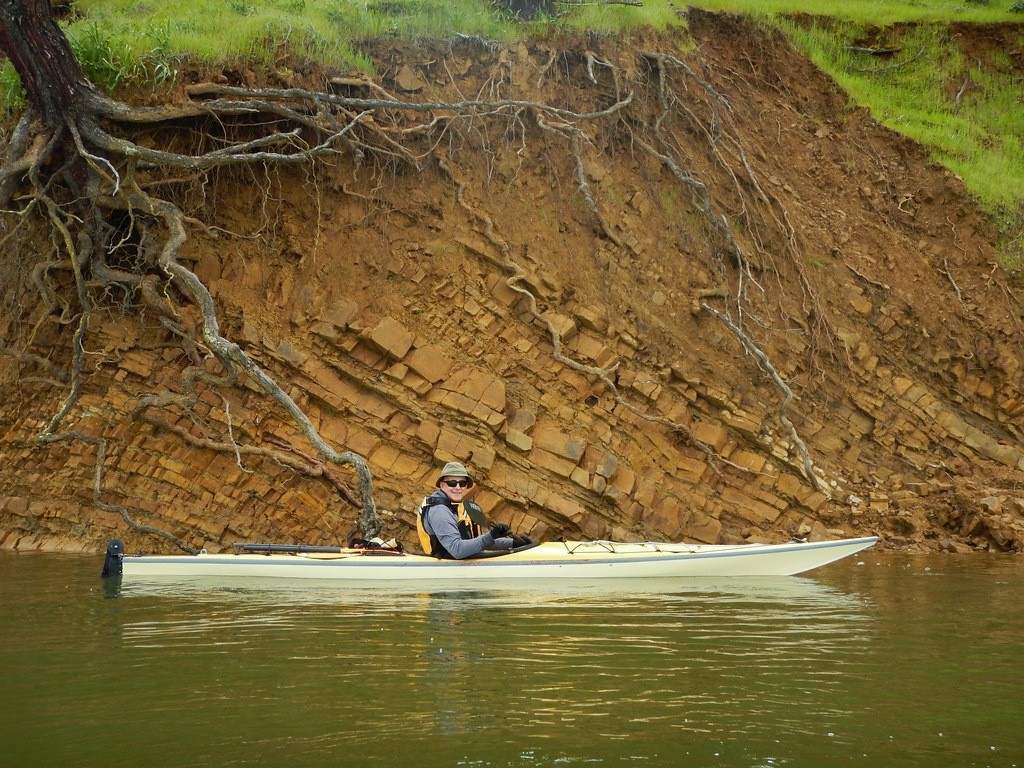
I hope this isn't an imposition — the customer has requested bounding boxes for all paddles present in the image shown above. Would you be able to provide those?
[233,542,401,559]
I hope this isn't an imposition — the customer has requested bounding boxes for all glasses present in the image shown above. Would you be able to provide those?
[442,479,468,487]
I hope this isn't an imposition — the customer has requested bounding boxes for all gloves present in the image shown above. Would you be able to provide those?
[513,536,532,549]
[490,522,512,539]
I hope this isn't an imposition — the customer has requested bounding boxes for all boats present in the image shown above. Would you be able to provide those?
[101,537,885,581]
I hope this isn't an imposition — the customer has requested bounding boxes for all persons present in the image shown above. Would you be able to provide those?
[417,462,533,560]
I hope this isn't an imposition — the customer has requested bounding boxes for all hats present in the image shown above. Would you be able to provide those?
[436,462,473,489]
